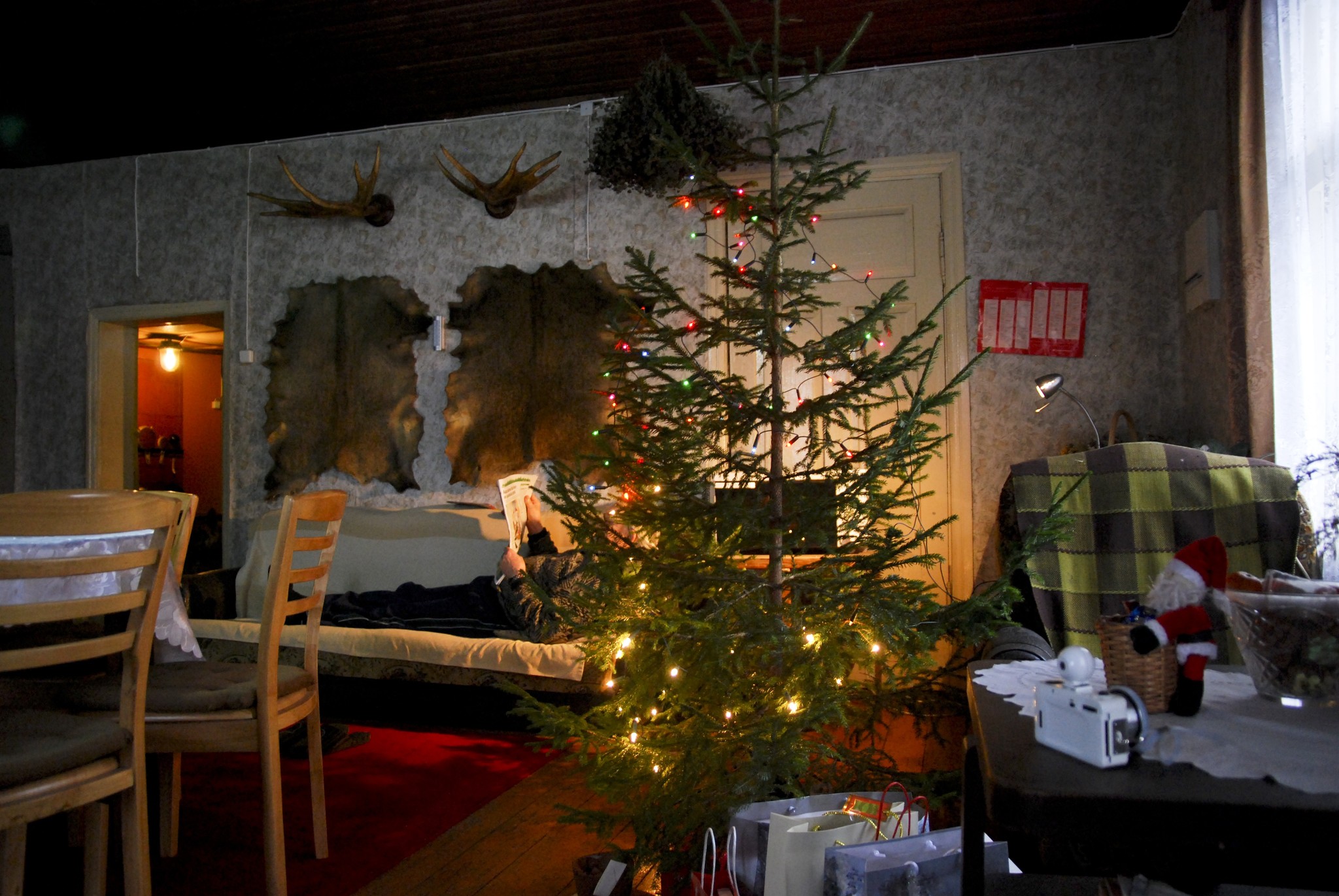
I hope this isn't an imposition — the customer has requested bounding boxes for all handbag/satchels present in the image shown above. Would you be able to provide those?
[824,824,1008,896]
[729,789,932,896]
[691,826,741,896]
[765,783,929,896]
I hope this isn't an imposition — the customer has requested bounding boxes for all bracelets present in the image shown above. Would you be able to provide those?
[511,574,521,579]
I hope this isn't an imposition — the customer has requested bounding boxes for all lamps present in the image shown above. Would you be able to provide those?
[157,339,183,373]
[1033,373,1100,449]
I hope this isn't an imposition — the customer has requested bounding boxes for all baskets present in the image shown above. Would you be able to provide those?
[1096,612,1178,712]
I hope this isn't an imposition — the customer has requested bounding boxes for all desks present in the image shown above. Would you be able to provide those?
[964,660,1339,896]
[0,531,202,665]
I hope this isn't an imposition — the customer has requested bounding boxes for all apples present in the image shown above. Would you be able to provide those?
[1225,571,1263,593]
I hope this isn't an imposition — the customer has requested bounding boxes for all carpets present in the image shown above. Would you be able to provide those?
[29,705,581,896]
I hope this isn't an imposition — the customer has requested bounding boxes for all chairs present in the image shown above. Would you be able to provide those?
[976,440,1323,666]
[0,490,345,896]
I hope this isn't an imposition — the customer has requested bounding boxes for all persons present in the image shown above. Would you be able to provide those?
[1128,534,1229,717]
[267,495,634,647]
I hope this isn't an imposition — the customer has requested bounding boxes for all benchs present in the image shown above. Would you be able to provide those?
[180,477,658,695]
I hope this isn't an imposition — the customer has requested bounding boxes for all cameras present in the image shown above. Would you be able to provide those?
[1033,643,1149,769]
